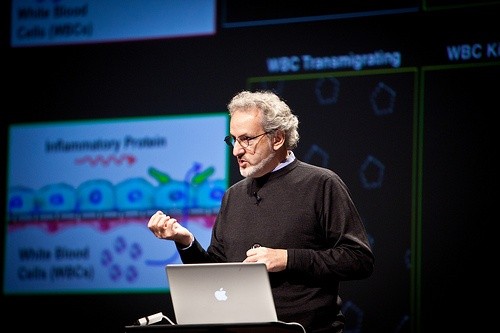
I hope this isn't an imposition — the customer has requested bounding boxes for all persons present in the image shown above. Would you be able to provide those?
[147,90,374,331]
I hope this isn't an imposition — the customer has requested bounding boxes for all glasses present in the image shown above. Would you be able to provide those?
[224,128,279,148]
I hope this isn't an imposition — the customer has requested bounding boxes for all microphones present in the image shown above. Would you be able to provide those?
[253,191,263,204]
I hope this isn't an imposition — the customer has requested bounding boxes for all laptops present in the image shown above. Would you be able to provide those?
[166,262,279,325]
